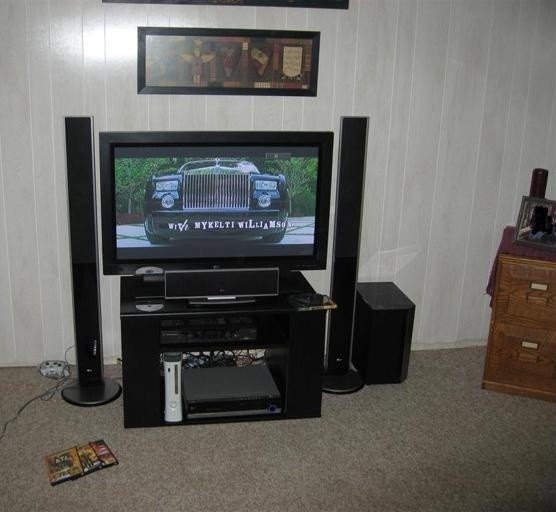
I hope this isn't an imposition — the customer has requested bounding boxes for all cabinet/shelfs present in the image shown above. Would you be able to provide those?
[482,227,556,402]
[120,272,325,428]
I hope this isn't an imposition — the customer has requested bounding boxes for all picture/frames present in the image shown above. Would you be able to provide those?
[512,196,556,252]
[137,26,320,97]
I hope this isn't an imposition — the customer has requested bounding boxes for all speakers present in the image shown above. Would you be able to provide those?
[62,115,122,408]
[322,116,371,396]
[164,267,281,305]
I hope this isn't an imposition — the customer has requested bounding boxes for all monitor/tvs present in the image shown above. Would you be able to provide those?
[98,129,334,300]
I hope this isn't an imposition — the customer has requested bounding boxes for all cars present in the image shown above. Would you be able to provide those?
[140,157,294,247]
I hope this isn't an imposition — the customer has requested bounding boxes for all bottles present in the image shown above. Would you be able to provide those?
[530,168,548,198]
